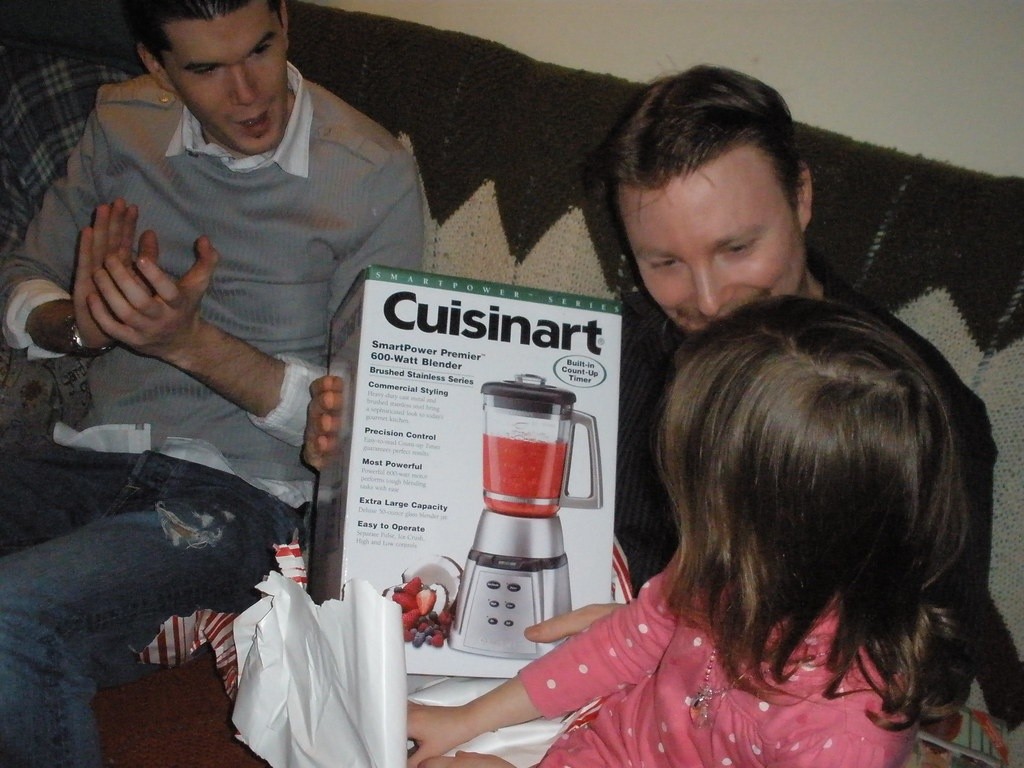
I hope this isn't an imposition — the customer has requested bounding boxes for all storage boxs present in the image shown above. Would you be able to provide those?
[307,263,624,677]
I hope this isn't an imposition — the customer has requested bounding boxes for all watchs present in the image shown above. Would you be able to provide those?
[66,315,119,354]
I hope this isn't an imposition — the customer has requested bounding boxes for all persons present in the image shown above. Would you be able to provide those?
[407,296,977,768]
[0,0,424,768]
[301,62,995,592]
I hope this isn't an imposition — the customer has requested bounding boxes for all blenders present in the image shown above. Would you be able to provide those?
[449,373,604,661]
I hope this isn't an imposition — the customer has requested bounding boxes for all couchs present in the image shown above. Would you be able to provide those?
[0,1,1024,768]
